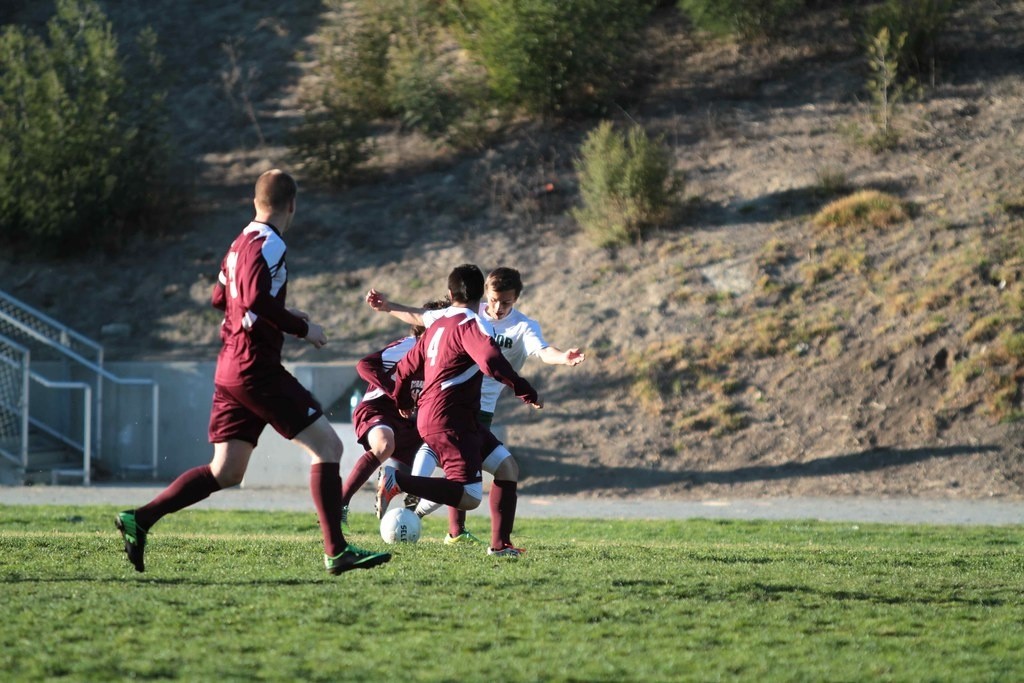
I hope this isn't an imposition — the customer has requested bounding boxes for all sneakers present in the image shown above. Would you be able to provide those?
[444,533,489,549]
[324,546,391,577]
[340,505,352,539]
[485,544,526,557]
[404,495,422,511]
[375,465,402,519]
[115,509,149,572]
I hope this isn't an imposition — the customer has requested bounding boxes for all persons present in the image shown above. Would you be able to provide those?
[340,296,450,523]
[364,268,585,518]
[114,169,393,577]
[375,263,544,561]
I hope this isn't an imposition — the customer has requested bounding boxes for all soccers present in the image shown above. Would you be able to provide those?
[379,507,422,544]
